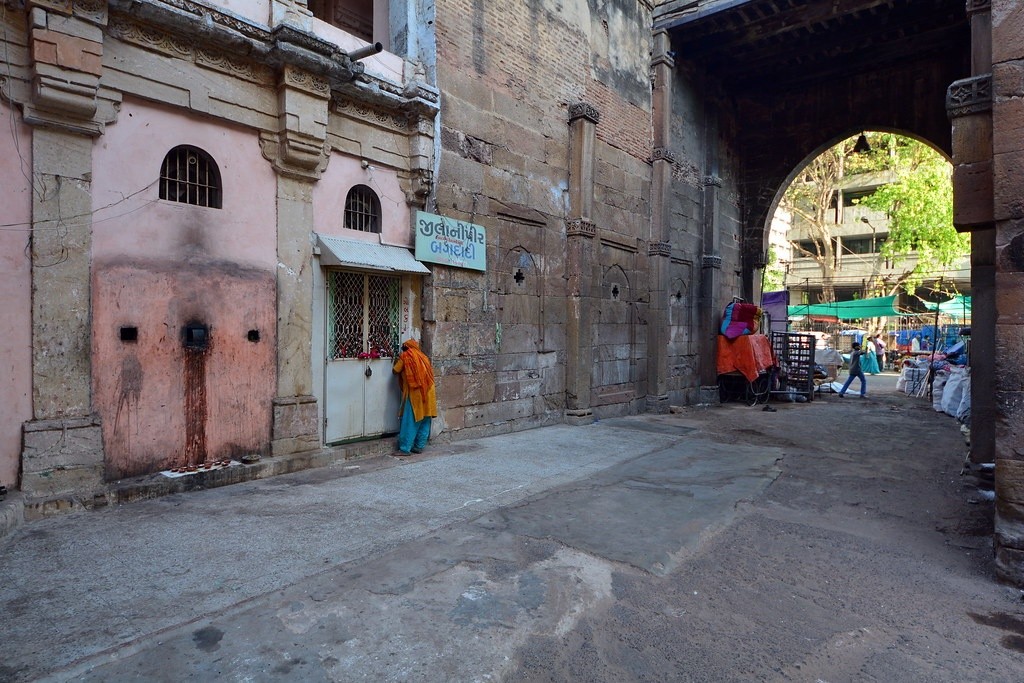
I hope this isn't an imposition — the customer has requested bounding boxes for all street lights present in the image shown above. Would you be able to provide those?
[861,216,876,298]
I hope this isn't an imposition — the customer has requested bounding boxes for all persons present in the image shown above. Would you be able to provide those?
[840,341,871,398]
[842,333,887,376]
[390,340,436,457]
[911,334,934,351]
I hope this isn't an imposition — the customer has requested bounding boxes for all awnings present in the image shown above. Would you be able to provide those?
[315,233,431,275]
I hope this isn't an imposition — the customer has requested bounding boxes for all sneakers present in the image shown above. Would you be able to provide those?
[392,450,411,456]
[860,394,869,398]
[411,448,422,454]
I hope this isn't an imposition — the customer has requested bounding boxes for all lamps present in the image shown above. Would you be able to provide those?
[854,131,871,154]
